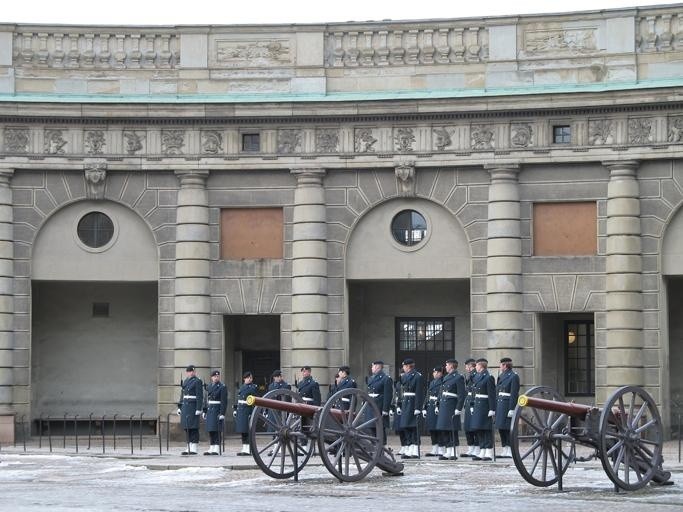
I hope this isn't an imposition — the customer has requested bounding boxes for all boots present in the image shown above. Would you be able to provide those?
[204,444,220,455]
[238,444,250,456]
[268,441,282,456]
[396,444,421,459]
[460,444,512,460]
[297,446,306,456]
[426,443,460,460]
[182,442,198,456]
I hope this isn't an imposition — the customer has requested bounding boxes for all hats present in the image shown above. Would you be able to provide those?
[300,367,310,372]
[465,358,488,363]
[272,371,281,378]
[186,365,195,371]
[372,361,384,365]
[210,371,220,376]
[501,358,512,362]
[402,359,415,365]
[243,372,252,378]
[336,366,349,378]
[433,359,458,372]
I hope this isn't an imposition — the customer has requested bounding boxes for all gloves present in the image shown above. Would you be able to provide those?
[382,407,515,417]
[195,410,206,420]
[233,411,237,418]
[177,409,181,415]
[219,415,224,421]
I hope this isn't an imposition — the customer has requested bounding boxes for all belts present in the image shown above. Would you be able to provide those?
[209,401,220,404]
[430,392,458,401]
[302,396,314,401]
[404,392,415,396]
[183,396,196,399]
[238,400,246,404]
[368,393,379,398]
[468,392,489,398]
[499,392,510,397]
[341,398,350,401]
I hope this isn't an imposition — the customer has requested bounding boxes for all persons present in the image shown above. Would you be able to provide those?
[231,371,261,457]
[363,360,394,445]
[328,371,340,394]
[494,357,520,458]
[176,365,203,455]
[469,358,497,462]
[398,358,424,460]
[201,370,228,455]
[293,365,322,457]
[420,365,447,456]
[394,367,408,456]
[458,358,482,458]
[328,365,356,457]
[265,369,291,457]
[433,358,465,461]
